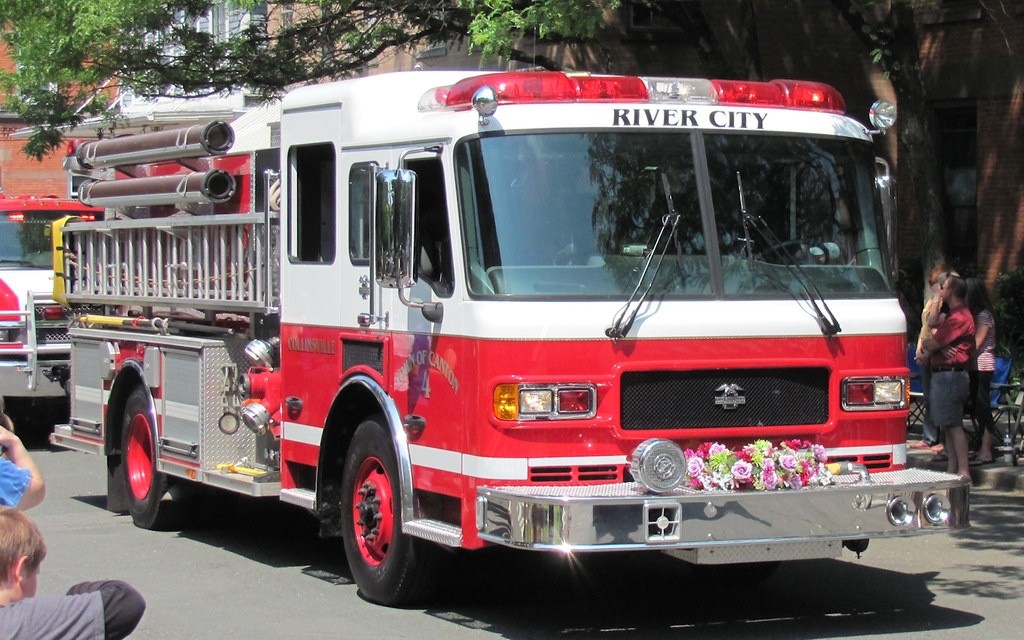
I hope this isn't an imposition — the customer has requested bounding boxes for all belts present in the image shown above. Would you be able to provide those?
[933,366,965,372]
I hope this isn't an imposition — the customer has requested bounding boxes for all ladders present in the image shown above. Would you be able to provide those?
[50,212,278,316]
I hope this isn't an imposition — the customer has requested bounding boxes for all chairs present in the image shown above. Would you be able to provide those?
[907,344,926,431]
[964,347,1013,445]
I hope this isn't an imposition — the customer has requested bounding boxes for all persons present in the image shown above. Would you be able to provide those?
[909,238,996,486]
[0,504,147,640]
[0,395,46,512]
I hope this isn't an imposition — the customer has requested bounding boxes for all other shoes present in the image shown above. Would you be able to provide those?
[910,441,996,466]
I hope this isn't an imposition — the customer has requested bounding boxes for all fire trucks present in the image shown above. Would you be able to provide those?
[46,69,971,603]
[0,196,106,417]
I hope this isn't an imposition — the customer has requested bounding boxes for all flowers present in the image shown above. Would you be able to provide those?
[683,438,832,492]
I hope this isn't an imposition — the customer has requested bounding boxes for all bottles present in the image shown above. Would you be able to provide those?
[1002,427,1015,466]
